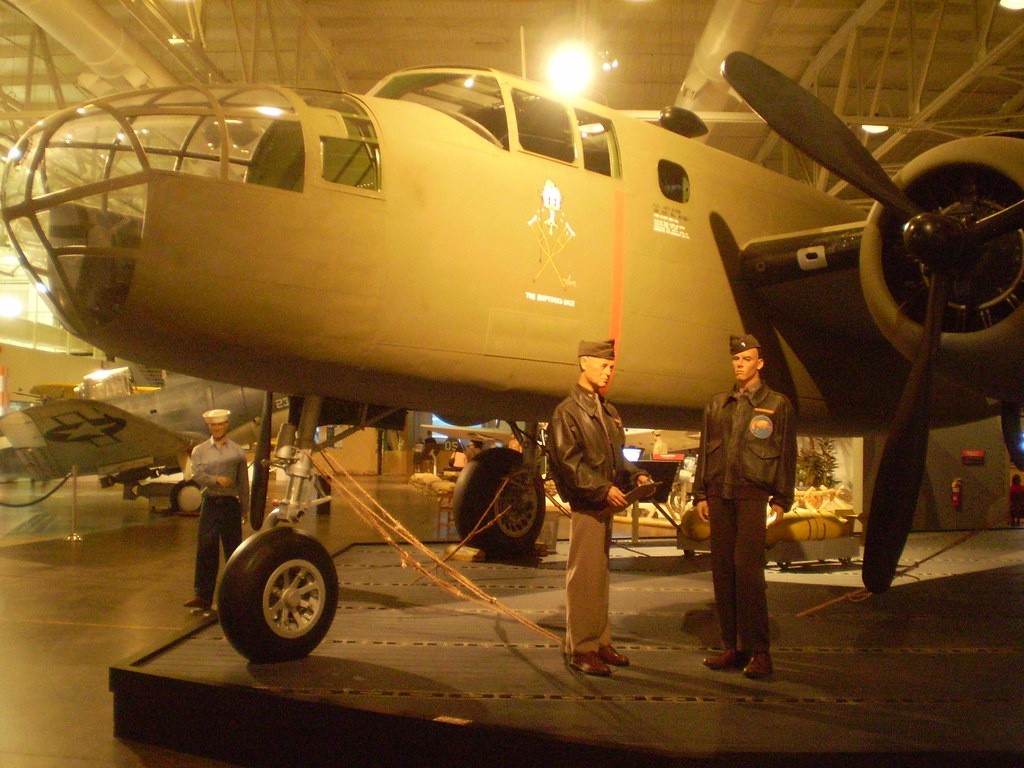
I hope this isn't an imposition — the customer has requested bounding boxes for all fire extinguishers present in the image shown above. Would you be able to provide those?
[952,478,963,508]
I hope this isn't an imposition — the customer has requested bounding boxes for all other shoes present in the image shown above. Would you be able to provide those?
[183,597,212,611]
[414,469,420,473]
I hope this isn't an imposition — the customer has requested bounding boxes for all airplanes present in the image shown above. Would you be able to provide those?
[0,50,1023,668]
[2,362,289,479]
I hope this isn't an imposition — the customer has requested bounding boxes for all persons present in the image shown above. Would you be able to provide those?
[183,410,250,610]
[423,430,483,470]
[547,339,655,676]
[691,334,797,679]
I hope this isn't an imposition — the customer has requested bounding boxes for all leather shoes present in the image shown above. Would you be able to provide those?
[600,644,629,666]
[570,651,612,675]
[703,647,744,669]
[743,652,773,678]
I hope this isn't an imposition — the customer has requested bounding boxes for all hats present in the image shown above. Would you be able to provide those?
[578,338,615,360]
[730,334,761,354]
[203,409,231,424]
